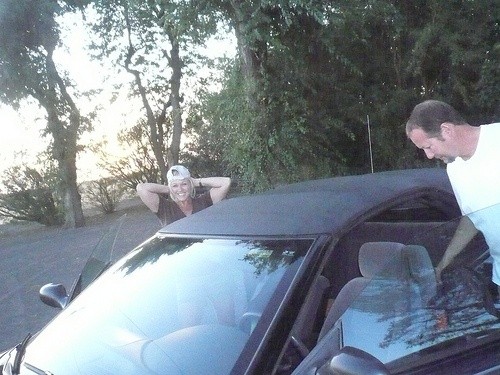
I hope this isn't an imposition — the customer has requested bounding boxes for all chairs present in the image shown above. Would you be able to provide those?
[316,242,420,359]
[251,267,329,375]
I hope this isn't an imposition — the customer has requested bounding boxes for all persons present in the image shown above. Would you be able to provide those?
[405,99,500,296]
[135,164,238,327]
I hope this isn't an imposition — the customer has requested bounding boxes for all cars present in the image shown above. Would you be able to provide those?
[0,166,500,375]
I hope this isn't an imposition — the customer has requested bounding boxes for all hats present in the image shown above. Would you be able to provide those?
[166,165,190,180]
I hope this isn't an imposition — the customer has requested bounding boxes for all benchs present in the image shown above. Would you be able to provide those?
[291,222,459,298]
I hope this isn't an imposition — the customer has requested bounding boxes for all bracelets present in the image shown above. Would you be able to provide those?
[199,178,202,187]
